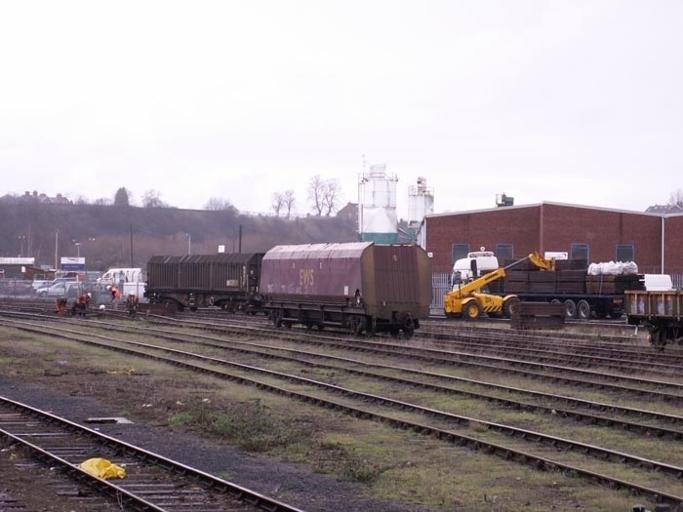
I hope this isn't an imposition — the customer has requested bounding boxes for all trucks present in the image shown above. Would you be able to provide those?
[98,267,142,287]
[621,289,682,349]
[448,248,645,319]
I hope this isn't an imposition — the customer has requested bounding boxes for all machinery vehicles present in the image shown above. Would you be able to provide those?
[442,254,555,318]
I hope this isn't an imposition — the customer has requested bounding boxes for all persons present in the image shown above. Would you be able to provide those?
[126,292,137,315]
[107,284,122,310]
[71,293,92,317]
[55,297,67,315]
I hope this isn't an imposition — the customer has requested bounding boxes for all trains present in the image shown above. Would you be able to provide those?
[144,241,434,336]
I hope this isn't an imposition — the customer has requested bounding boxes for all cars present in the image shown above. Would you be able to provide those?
[31,278,83,296]
